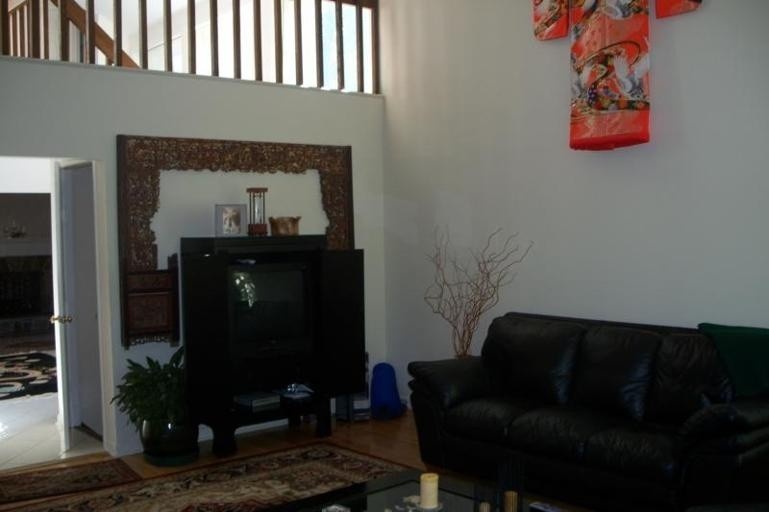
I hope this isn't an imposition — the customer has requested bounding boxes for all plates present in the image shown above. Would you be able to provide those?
[414,502,444,511]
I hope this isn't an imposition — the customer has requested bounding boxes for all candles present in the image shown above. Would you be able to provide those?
[419,472,440,511]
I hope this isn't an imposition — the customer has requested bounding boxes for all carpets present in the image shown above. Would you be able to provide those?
[0,456,145,505]
[0,439,414,512]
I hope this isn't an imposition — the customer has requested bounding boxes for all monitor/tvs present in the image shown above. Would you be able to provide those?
[179,235,327,377]
[214,249,365,395]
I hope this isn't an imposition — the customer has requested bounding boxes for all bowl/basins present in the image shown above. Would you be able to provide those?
[268,214,304,235]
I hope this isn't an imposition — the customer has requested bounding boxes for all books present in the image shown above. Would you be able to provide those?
[235,391,281,406]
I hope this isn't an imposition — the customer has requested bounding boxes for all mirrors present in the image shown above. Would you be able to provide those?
[116,133,355,348]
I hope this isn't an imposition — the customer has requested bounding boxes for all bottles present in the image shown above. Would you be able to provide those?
[419,472,439,507]
[476,490,519,512]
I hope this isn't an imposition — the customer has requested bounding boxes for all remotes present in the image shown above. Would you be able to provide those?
[529,501,561,512]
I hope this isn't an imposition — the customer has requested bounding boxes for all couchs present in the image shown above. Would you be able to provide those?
[407,311,738,512]
[696,321,769,479]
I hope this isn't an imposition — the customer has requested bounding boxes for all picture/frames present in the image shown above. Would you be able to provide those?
[215,203,248,238]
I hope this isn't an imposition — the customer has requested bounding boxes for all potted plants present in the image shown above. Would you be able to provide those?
[109,341,205,468]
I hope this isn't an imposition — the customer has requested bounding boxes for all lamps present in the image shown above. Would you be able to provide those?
[2,217,28,240]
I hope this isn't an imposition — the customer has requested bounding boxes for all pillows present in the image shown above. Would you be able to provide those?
[598,356,658,427]
[511,322,588,411]
